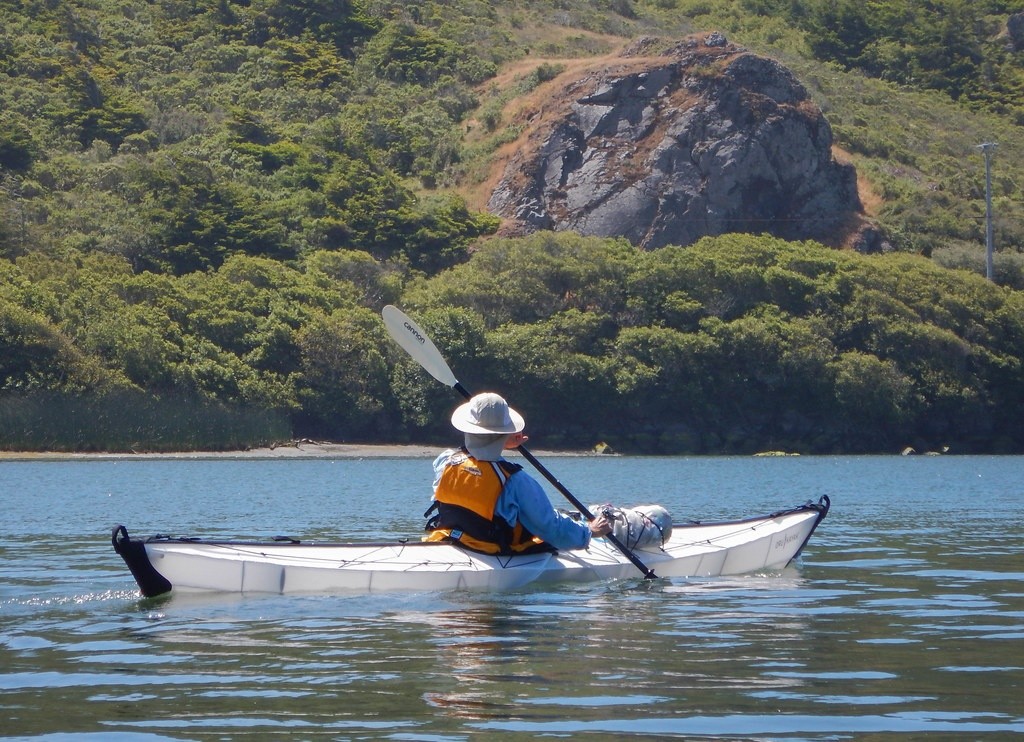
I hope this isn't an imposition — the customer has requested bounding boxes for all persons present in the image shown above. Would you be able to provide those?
[421,393,613,557]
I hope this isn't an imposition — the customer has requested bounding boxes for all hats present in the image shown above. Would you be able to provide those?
[451,393,525,434]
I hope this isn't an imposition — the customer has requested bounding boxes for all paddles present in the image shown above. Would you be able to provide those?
[382,302,660,581]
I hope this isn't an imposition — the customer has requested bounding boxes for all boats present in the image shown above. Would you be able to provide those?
[106,490,834,600]
